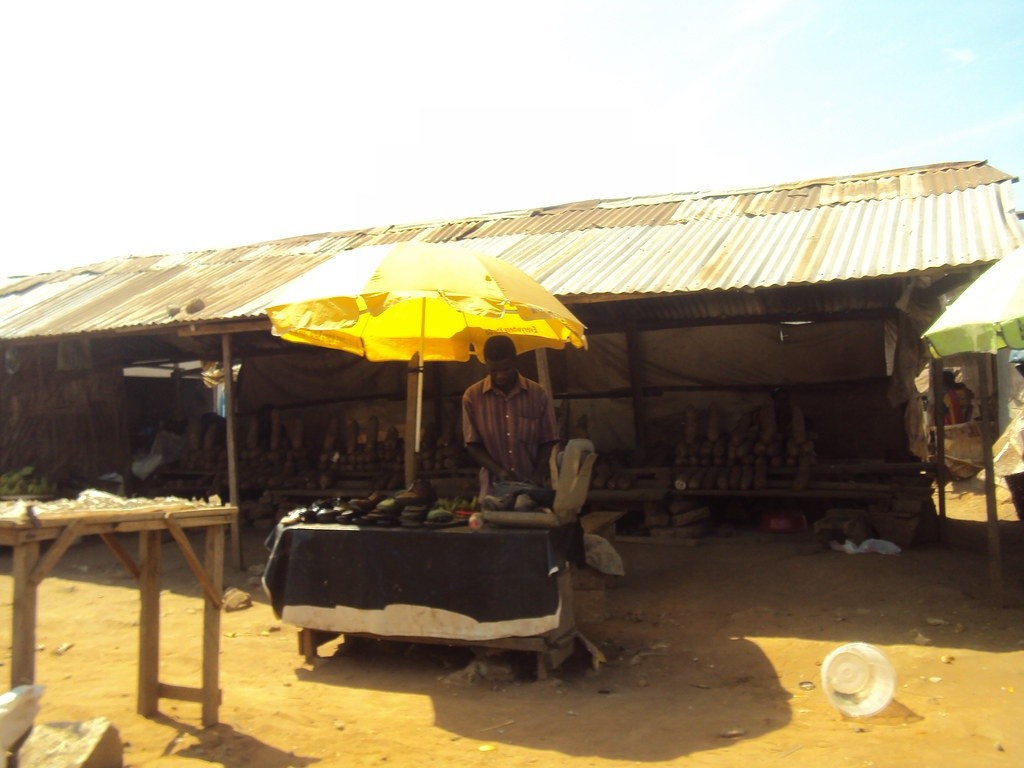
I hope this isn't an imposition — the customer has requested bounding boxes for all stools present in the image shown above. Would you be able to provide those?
[813,509,868,548]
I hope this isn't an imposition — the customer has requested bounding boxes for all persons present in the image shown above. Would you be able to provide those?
[922,370,975,425]
[463,335,560,511]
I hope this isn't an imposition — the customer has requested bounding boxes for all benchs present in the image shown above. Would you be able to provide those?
[581,506,624,579]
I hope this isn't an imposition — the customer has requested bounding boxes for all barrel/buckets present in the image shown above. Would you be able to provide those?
[821,641,896,718]
[949,405,967,424]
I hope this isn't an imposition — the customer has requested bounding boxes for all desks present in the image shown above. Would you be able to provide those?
[0,491,241,728]
[284,512,588,680]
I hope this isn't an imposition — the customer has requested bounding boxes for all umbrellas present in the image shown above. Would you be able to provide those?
[265,241,587,493]
[921,245,1024,359]
